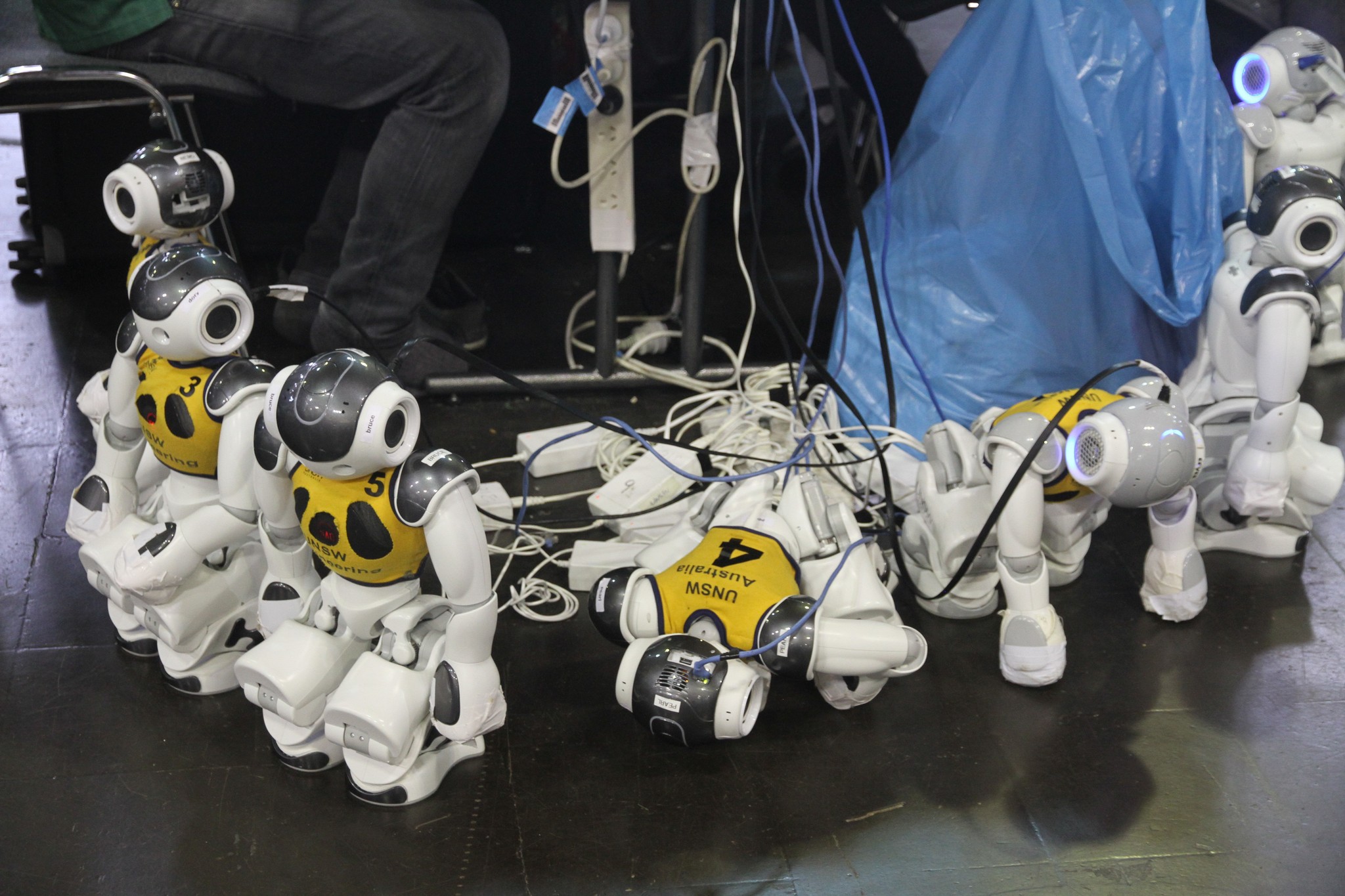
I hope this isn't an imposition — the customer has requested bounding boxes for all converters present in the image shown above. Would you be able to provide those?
[473,482,514,533]
[855,443,923,515]
[515,419,632,478]
[568,444,704,592]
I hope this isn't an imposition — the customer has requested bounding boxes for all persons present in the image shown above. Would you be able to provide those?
[1181,165,1345,559]
[912,371,1209,688]
[63,133,506,811]
[1226,20,1345,364]
[32,2,512,388]
[583,446,924,744]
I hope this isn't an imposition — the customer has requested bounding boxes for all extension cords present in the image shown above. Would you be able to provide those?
[582,1,635,252]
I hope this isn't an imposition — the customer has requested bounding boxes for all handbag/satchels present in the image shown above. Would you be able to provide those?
[830,1,1241,463]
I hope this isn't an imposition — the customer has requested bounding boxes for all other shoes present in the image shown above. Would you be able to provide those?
[310,316,468,398]
[268,290,486,350]
